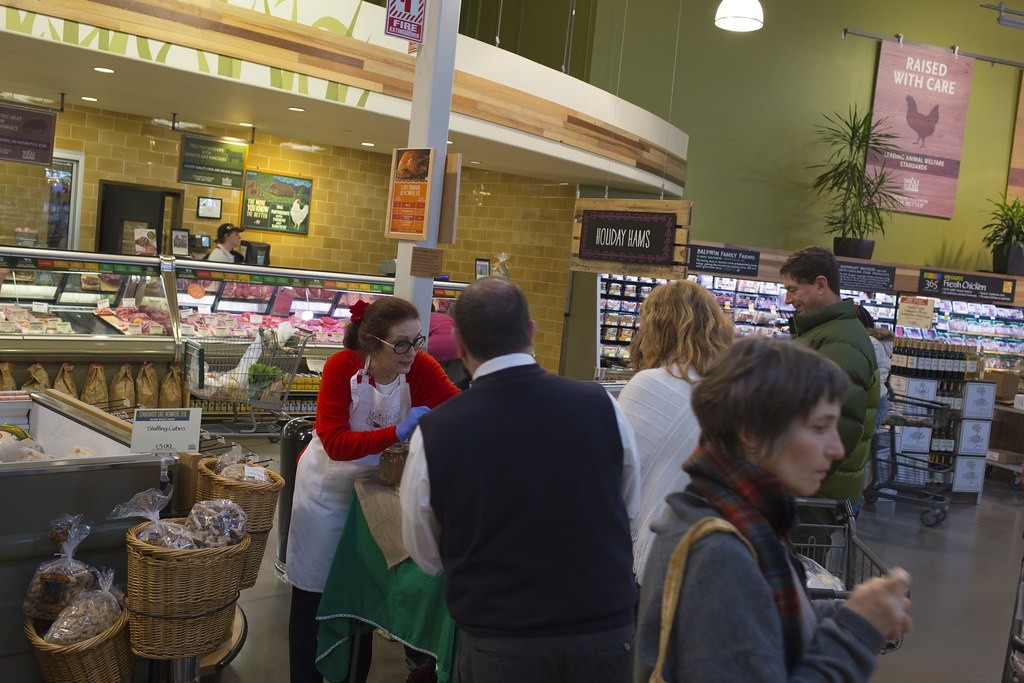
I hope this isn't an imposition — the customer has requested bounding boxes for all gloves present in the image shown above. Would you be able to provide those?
[396,405,434,441]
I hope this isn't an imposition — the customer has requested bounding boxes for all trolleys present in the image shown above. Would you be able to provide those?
[178,326,318,442]
[791,497,887,590]
[866,393,960,528]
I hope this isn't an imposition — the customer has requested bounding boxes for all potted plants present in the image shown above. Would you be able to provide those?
[982,189,1024,277]
[804,100,916,260]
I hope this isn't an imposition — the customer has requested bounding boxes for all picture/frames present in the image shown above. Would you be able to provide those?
[474,258,490,280]
[170,226,190,257]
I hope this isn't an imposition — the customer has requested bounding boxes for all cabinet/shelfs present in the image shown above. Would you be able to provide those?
[182,388,318,420]
[984,403,1024,491]
[875,376,997,503]
[894,306,1024,373]
[599,276,899,372]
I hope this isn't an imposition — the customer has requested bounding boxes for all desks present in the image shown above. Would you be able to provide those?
[314,479,464,683]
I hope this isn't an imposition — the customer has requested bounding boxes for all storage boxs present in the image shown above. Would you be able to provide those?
[984,368,1024,465]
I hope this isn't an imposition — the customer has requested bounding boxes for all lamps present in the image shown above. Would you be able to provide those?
[714,0,764,31]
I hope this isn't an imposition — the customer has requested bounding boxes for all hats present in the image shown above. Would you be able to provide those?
[217,223,244,237]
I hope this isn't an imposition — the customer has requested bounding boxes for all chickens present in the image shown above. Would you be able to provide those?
[290,198,308,229]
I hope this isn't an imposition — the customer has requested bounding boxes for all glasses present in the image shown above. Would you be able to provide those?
[373,332,426,355]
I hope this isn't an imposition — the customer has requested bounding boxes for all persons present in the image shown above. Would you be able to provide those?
[207,223,245,263]
[616,281,739,577]
[282,297,461,683]
[780,246,894,567]
[635,335,913,683]
[398,279,639,683]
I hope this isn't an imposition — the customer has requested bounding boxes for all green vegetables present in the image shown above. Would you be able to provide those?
[247,363,283,401]
